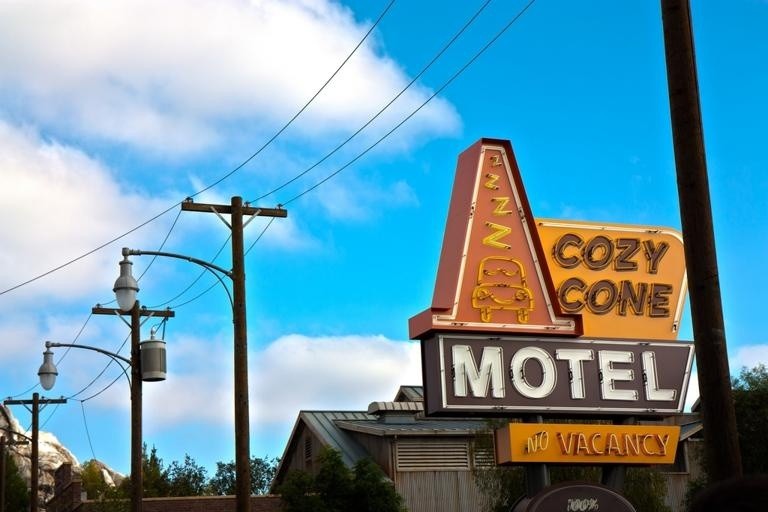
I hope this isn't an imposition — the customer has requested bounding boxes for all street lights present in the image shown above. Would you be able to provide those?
[38,339,142,512]
[112,246,252,511]
